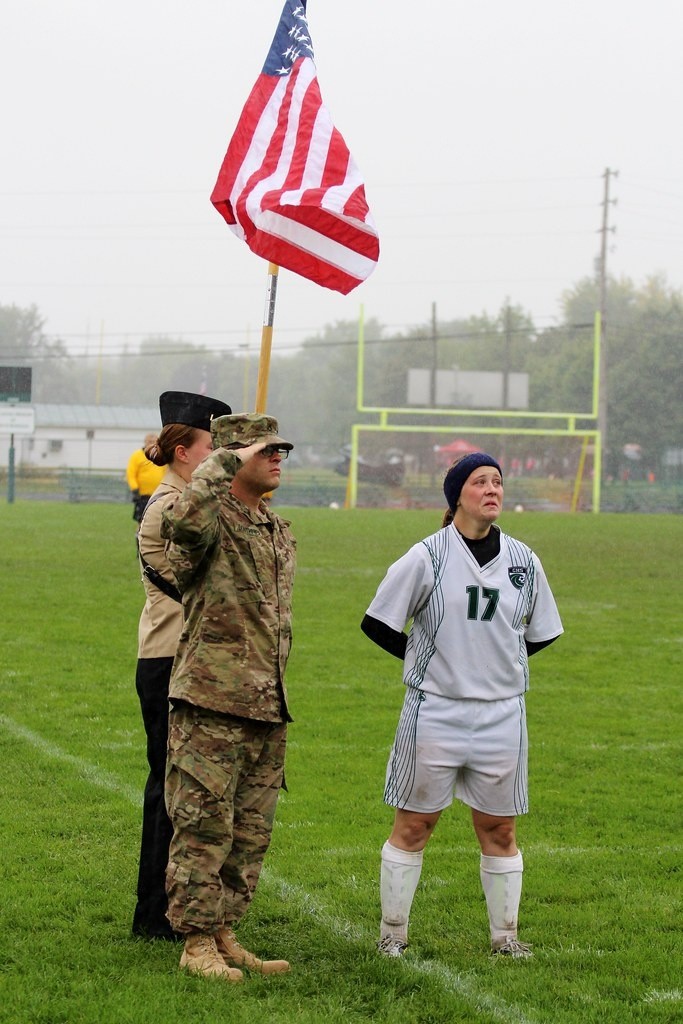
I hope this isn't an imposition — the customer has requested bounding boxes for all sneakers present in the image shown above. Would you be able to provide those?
[375,936,410,960]
[215,926,293,979]
[490,941,534,961]
[180,934,246,985]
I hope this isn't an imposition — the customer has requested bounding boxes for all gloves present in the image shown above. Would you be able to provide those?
[132,488,142,503]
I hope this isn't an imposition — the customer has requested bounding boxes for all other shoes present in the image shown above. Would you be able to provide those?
[130,902,182,942]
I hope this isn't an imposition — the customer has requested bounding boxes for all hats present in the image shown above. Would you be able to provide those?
[158,390,232,431]
[209,411,295,453]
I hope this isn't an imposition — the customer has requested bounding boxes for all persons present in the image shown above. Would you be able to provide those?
[132,392,231,940]
[160,414,298,984]
[495,440,682,480]
[361,453,566,958]
[129,435,167,557]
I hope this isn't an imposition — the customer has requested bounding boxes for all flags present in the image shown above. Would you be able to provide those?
[210,0,380,297]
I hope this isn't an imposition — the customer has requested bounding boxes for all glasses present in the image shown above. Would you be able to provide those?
[261,444,291,459]
